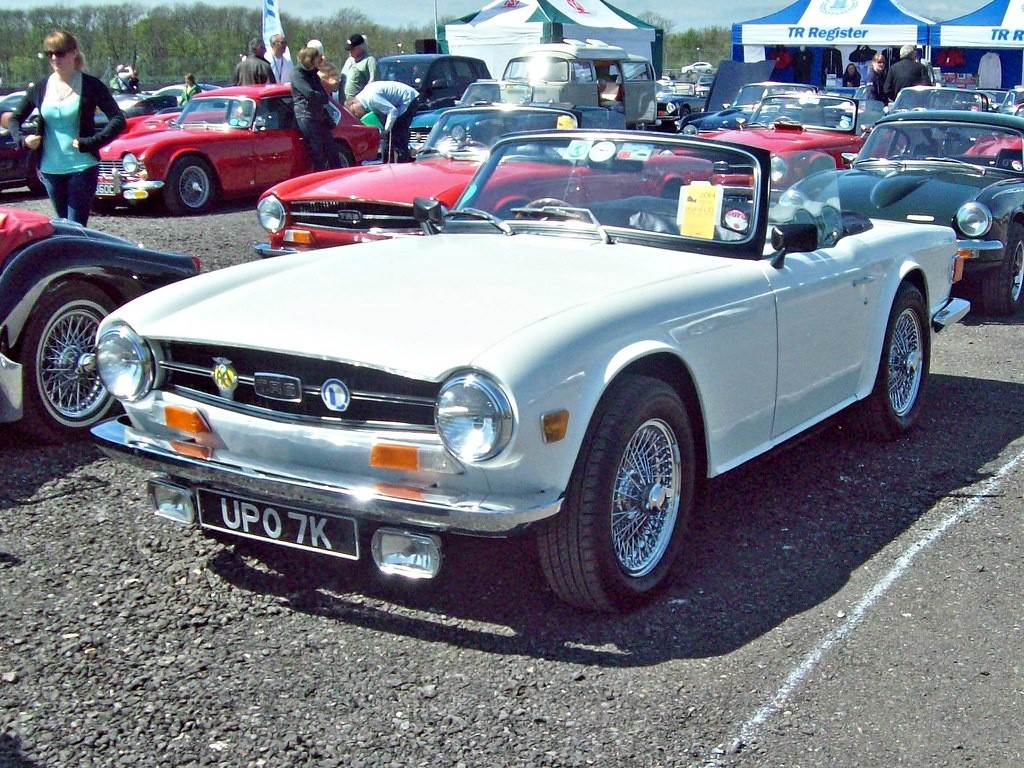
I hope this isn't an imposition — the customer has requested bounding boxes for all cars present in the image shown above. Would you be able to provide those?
[0,206,202,440]
[0,85,716,256]
[89,127,971,615]
[678,80,1024,317]
[656,74,715,131]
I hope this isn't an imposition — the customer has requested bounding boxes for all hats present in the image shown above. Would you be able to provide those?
[344,35,364,50]
[116,64,124,72]
[307,39,323,56]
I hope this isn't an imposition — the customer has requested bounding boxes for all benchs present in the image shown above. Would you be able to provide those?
[592,196,679,227]
[599,82,623,109]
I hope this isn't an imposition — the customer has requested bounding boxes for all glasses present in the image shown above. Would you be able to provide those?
[45,48,69,59]
[275,41,287,46]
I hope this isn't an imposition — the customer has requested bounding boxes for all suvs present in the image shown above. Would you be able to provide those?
[377,54,492,113]
[682,62,713,74]
[473,38,657,133]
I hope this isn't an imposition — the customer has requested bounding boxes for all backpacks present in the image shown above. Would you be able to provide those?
[110,76,121,92]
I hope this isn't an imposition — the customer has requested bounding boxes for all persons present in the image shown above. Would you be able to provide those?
[338,34,378,105]
[8,31,127,229]
[344,81,421,163]
[290,47,341,172]
[264,34,293,84]
[669,71,675,80]
[884,45,932,100]
[234,38,276,87]
[843,64,861,87]
[116,64,139,94]
[865,54,889,106]
[307,39,340,94]
[178,73,202,107]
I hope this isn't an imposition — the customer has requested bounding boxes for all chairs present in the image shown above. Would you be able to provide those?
[801,105,826,126]
[912,143,939,159]
[856,112,882,135]
[995,147,1024,170]
[470,117,510,144]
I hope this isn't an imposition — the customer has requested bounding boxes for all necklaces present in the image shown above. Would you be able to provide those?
[56,72,77,100]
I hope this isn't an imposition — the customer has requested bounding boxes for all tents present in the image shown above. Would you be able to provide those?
[730,0,1024,89]
[437,0,664,79]
